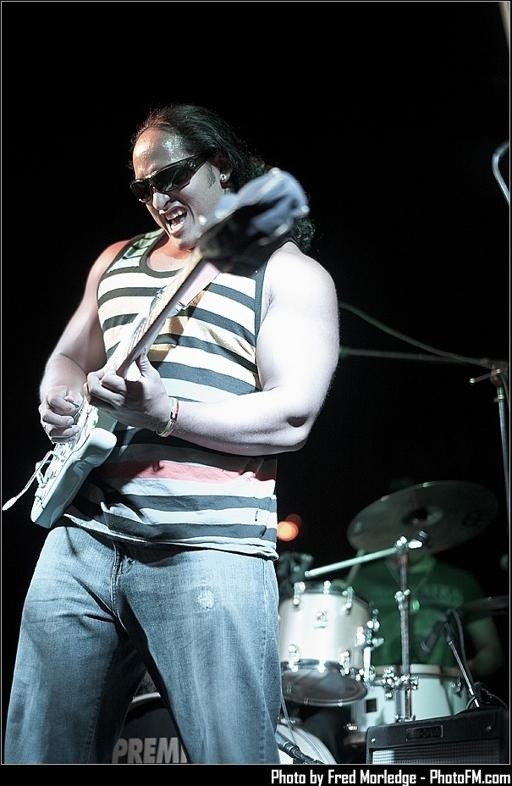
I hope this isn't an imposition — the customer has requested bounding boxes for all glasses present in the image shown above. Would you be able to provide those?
[130,152,209,202]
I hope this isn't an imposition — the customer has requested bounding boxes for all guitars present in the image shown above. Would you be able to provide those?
[31,167,310,527]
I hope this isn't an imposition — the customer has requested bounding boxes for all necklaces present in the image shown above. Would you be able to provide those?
[384,559,432,613]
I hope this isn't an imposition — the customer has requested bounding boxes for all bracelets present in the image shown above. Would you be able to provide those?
[156,397,178,437]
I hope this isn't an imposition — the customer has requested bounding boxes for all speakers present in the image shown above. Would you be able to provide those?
[364,707,509,764]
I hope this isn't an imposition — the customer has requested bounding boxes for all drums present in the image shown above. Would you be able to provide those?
[277,579,380,707]
[113,691,337,765]
[342,664,474,749]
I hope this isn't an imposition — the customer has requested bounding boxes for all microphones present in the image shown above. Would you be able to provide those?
[274,730,299,758]
[414,612,451,663]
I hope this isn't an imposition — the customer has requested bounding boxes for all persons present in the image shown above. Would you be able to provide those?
[303,552,504,764]
[5,105,339,765]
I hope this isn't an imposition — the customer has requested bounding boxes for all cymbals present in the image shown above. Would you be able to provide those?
[460,595,509,610]
[348,480,487,554]
